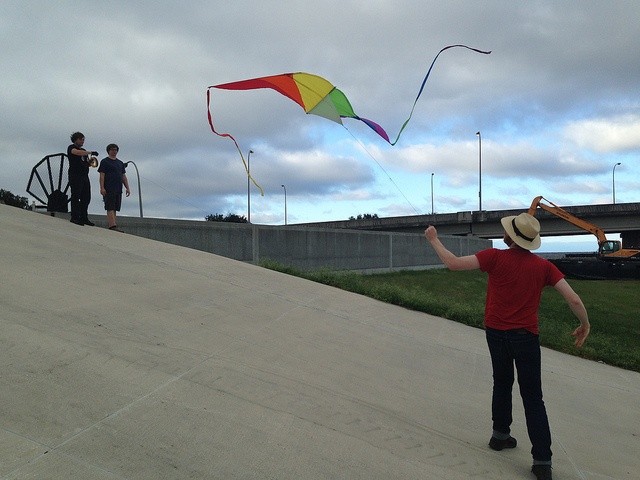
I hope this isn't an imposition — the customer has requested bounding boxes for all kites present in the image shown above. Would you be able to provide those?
[206,44,492,196]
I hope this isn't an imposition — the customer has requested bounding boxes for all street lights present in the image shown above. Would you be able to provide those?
[613,163,621,204]
[248,151,253,223]
[124,161,143,217]
[282,185,286,225]
[476,132,481,212]
[431,173,434,216]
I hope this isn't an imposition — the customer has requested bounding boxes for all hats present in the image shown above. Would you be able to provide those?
[501,213,541,250]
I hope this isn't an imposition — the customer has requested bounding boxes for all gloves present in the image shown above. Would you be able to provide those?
[90,152,99,156]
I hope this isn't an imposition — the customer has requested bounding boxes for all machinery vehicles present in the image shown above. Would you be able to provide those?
[527,196,640,280]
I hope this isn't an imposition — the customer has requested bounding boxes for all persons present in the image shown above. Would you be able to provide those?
[67,132,99,226]
[97,144,131,233]
[423,212,591,480]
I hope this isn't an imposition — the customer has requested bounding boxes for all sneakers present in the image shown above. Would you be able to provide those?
[118,230,125,233]
[70,219,84,226]
[489,436,517,451]
[531,464,553,479]
[85,221,96,226]
[109,226,118,229]
